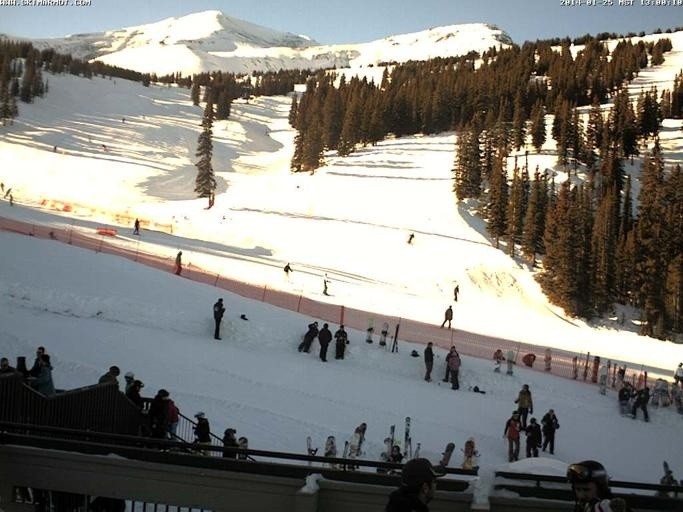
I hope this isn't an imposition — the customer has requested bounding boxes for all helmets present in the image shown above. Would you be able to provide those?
[567,461,608,502]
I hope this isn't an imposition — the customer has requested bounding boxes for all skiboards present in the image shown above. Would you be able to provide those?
[392,323,400,352]
[611,352,647,401]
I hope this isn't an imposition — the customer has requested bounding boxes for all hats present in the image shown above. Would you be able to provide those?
[401,458,447,481]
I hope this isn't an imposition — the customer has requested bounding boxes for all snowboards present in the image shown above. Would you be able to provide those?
[379,322,388,351]
[366,317,376,344]
[493,350,502,373]
[303,415,455,484]
[544,348,552,372]
[507,351,513,374]
[572,351,608,395]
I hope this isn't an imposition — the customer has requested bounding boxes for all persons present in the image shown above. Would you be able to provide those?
[446,351,462,389]
[212,297,226,340]
[631,386,650,422]
[564,460,627,511]
[284,262,293,277]
[318,323,332,362]
[387,446,402,464]
[334,324,350,360]
[2,341,252,461]
[673,363,683,385]
[133,218,139,235]
[540,409,558,455]
[524,418,542,457]
[175,251,183,275]
[323,272,330,296]
[298,321,318,353]
[453,285,459,302]
[381,458,449,510]
[501,411,522,462]
[439,304,453,329]
[440,345,459,382]
[349,422,367,459]
[513,384,534,431]
[424,341,435,381]
[617,382,633,417]
[460,440,481,474]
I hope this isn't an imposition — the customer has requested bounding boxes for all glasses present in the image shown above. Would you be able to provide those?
[567,464,592,482]
